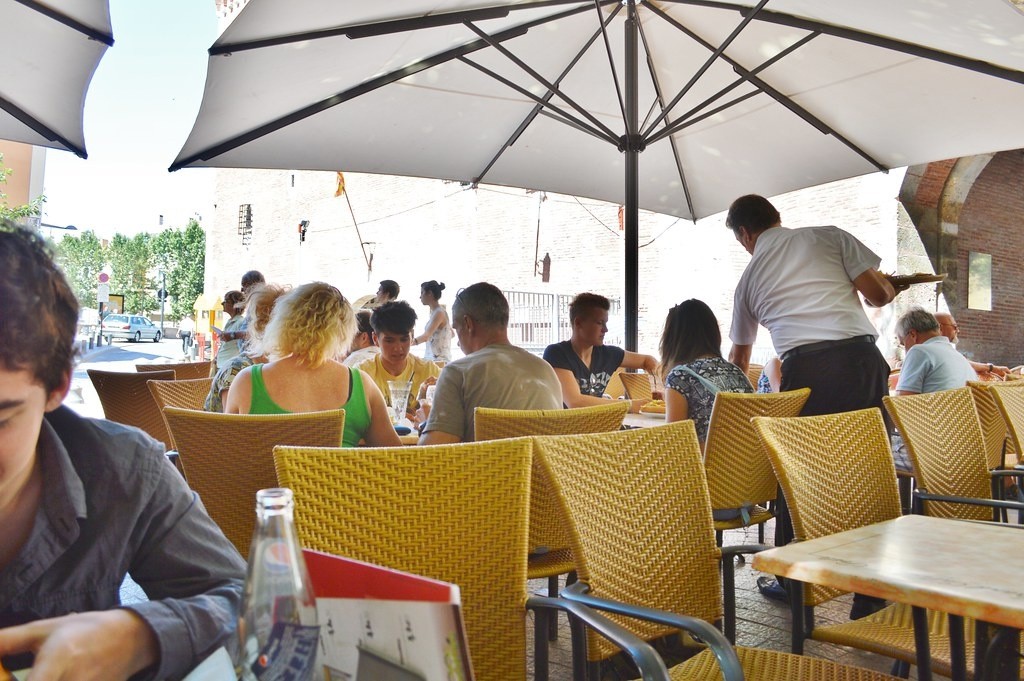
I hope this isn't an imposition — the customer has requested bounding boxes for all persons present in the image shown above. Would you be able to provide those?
[202,270,453,413]
[0,216,248,681]
[652,298,757,522]
[889,307,1012,473]
[725,193,912,621]
[175,312,196,355]
[543,292,656,414]
[224,280,404,447]
[416,282,564,561]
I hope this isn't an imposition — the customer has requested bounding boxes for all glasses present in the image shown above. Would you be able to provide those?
[224,299,231,303]
[939,322,957,330]
[456,288,480,323]
[898,329,919,350]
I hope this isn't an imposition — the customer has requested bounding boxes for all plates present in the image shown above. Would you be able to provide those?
[639,410,666,419]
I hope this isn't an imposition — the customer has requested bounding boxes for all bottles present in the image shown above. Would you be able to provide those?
[236,487,324,681]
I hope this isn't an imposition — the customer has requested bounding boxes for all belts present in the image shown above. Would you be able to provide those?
[779,335,874,363]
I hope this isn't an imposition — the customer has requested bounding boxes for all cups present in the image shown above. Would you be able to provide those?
[418,399,433,421]
[387,380,413,421]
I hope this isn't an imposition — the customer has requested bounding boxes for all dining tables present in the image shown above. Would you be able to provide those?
[360,414,420,452]
[622,412,668,428]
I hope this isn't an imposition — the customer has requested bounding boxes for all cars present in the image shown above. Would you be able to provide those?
[101,314,162,343]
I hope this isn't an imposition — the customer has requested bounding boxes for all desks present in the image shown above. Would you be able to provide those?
[751,514,1024,681]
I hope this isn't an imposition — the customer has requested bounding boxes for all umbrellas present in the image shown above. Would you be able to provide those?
[0,0,115,159]
[166,0,1024,403]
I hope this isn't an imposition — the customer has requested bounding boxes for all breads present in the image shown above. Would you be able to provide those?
[422,403,431,419]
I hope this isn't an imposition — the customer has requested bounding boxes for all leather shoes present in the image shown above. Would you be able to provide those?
[757,576,788,602]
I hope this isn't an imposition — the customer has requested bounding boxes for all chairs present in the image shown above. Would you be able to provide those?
[89,356,1024,681]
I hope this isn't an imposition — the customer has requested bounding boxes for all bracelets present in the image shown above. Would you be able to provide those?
[987,362,993,372]
